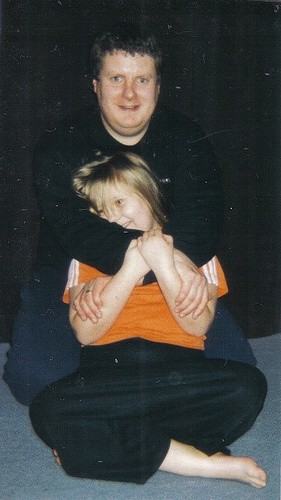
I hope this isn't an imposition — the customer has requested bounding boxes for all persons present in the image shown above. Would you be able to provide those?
[3,28,260,405]
[27,148,270,488]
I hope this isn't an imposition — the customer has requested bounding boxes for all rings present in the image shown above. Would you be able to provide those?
[85,290,93,294]
